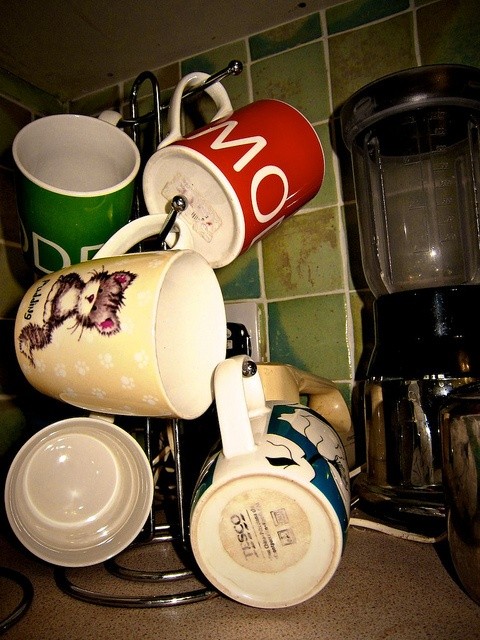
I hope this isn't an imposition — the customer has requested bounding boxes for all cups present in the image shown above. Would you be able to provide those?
[14,211,228,419]
[6,405,156,571]
[183,348,353,609]
[140,68,328,269]
[9,109,141,275]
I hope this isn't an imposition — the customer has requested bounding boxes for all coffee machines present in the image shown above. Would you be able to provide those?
[324,64,480,539]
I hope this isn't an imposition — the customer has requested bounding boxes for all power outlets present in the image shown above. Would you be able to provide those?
[225,302,268,365]
